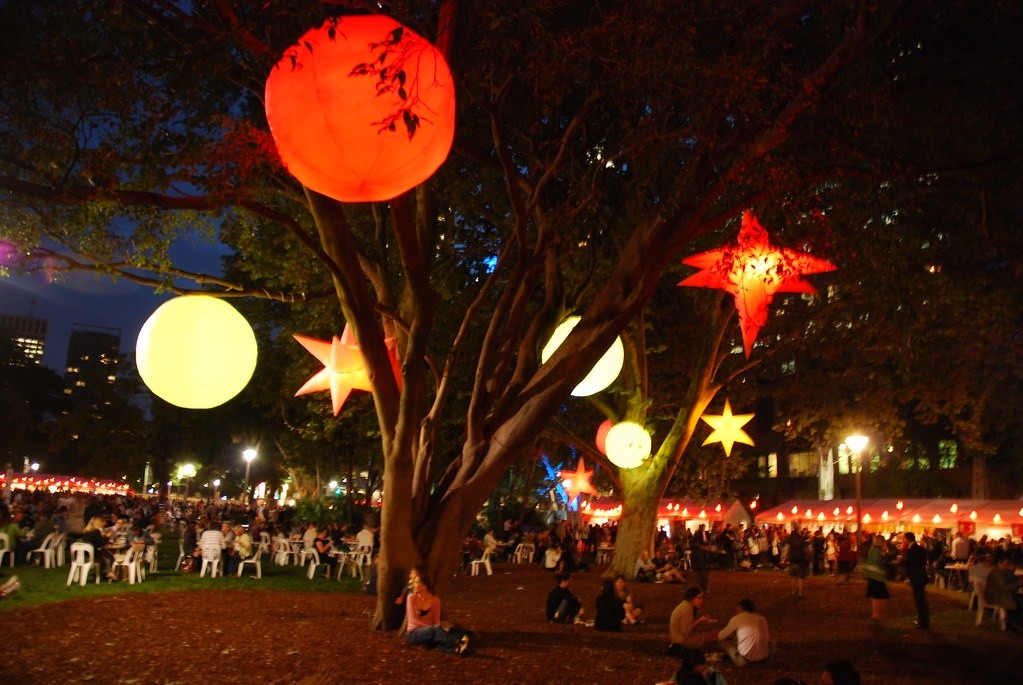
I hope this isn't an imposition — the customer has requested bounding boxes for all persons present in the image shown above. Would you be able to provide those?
[676,653,716,684]
[664,586,725,661]
[0,488,1023,634]
[546,571,588,625]
[715,598,771,667]
[396,565,475,653]
[597,574,646,628]
[820,662,860,685]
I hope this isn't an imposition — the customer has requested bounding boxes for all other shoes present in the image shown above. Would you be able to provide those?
[455,635,471,654]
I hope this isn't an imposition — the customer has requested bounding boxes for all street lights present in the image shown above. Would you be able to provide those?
[242,447,256,505]
[844,433,870,549]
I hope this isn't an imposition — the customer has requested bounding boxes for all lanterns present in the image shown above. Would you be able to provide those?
[136,297,258,409]
[542,316,625,397]
[265,16,454,204]
[595,418,651,468]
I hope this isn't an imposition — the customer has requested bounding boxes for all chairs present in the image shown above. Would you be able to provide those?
[471,547,494,576]
[973,582,1007,629]
[0,530,376,587]
[512,544,536,564]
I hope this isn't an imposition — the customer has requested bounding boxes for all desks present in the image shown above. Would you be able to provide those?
[942,564,970,591]
